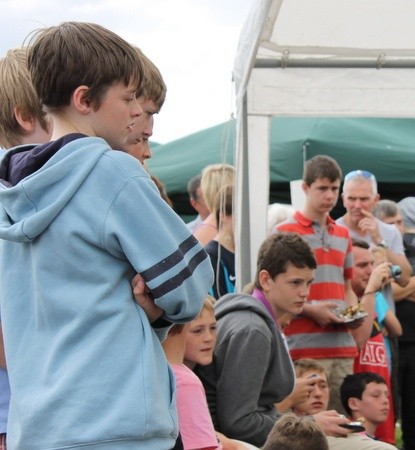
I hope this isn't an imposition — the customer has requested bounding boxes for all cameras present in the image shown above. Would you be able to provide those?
[390,265,402,279]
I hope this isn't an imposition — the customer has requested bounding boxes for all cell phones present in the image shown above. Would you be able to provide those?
[305,373,320,379]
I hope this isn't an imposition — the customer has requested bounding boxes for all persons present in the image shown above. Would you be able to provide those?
[261,416,330,450]
[188,155,415,450]
[163,294,224,450]
[0,17,215,449]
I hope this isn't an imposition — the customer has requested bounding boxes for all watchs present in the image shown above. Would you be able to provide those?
[377,241,385,248]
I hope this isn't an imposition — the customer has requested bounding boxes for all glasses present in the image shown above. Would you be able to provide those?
[345,170,372,179]
